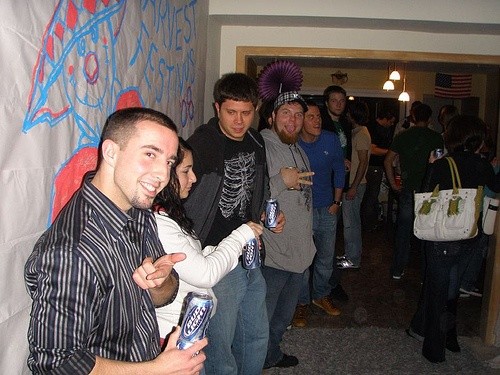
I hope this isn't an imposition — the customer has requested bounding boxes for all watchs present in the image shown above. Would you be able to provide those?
[334,201,342,206]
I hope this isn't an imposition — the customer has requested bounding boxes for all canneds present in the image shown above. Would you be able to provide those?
[433,148,442,159]
[264,198,280,228]
[242,238,261,269]
[177,291,213,356]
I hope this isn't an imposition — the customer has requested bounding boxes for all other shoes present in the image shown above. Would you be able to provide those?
[293,303,310,327]
[311,295,341,317]
[334,253,348,261]
[263,354,299,371]
[333,257,358,270]
[393,270,407,280]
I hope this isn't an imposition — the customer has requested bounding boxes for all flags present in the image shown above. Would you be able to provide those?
[434,73,472,98]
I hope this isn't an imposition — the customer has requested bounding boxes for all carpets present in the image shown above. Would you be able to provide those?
[268,326,495,375]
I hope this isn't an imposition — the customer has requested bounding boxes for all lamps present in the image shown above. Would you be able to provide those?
[382,63,411,103]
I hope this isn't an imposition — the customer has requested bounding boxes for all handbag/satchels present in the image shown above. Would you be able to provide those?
[480,196,499,237]
[410,153,483,242]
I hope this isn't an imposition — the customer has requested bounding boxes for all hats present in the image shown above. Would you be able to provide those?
[271,90,310,115]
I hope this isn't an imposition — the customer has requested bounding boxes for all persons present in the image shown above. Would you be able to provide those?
[408,113,500,364]
[359,107,397,232]
[393,101,432,138]
[152,137,264,350]
[335,100,371,268]
[320,86,352,300]
[384,104,444,279]
[439,105,458,139]
[260,91,317,369]
[295,100,345,325]
[24,107,209,375]
[184,73,287,375]
